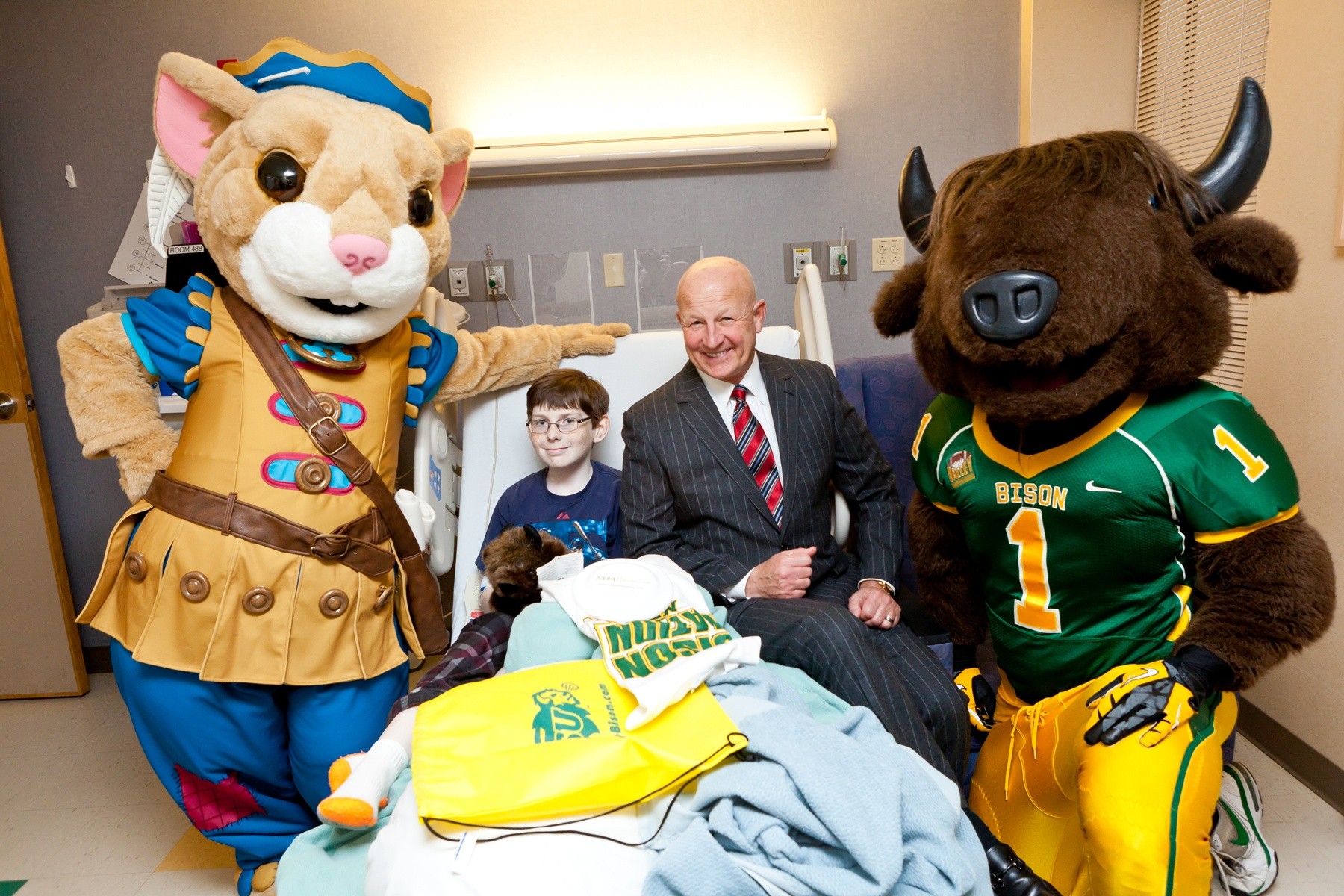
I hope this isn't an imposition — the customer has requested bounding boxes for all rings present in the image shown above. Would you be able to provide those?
[885,615,895,625]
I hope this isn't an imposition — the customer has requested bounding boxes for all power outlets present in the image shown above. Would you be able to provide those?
[871,238,906,273]
[487,263,506,294]
[448,266,470,300]
[828,245,851,274]
[794,248,811,276]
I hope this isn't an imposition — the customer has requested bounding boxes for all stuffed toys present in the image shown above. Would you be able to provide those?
[875,78,1333,895]
[315,519,568,831]
[52,38,631,895]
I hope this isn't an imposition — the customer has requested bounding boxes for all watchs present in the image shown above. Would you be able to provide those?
[878,579,894,593]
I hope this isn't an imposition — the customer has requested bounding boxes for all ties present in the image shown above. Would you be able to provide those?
[731,383,784,530]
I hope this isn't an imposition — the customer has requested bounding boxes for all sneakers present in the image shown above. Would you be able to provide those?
[1210,761,1280,896]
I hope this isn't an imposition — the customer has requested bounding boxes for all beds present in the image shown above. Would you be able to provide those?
[276,260,854,653]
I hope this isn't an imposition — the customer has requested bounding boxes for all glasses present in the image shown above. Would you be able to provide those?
[526,417,593,434]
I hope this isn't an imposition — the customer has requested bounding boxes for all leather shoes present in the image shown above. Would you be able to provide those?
[986,844,1065,896]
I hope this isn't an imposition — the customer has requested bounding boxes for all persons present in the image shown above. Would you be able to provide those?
[621,258,1061,896]
[476,369,623,615]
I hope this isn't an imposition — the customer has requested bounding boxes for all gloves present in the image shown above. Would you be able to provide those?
[1083,660,1205,750]
[951,666,998,751]
